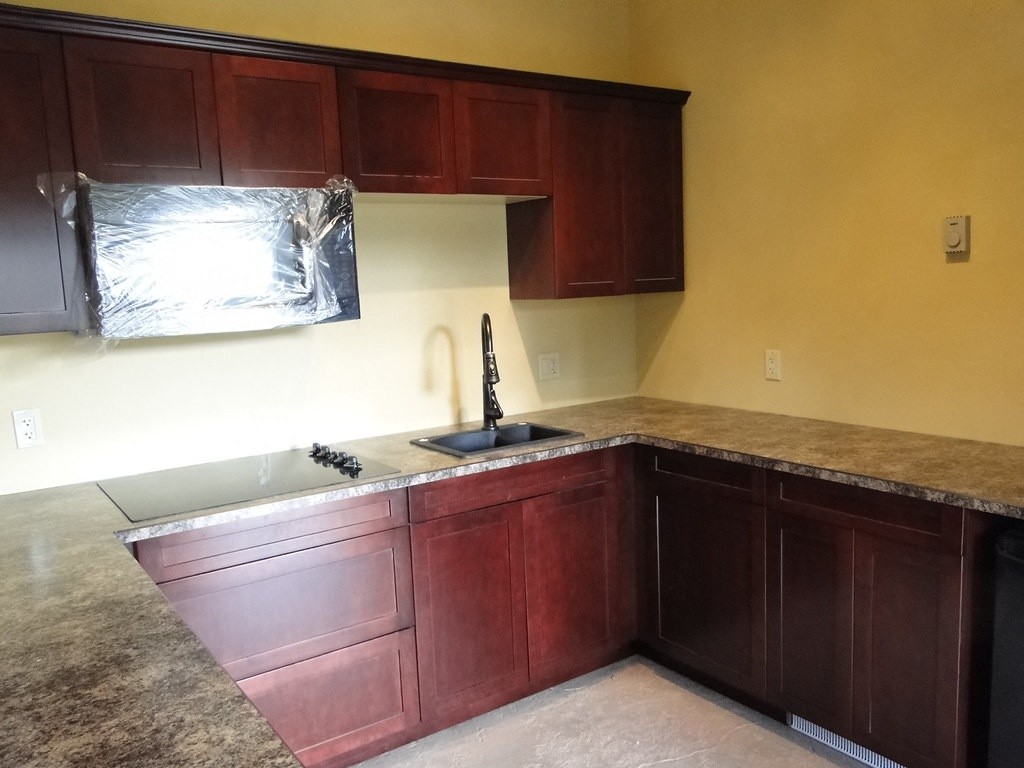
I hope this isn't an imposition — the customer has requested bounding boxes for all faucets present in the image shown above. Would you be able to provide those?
[481,313,503,429]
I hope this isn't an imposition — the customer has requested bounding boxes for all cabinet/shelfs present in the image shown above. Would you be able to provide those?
[335,66,551,204]
[639,444,995,768]
[135,487,419,766]
[507,92,686,298]
[409,446,623,725]
[63,34,344,191]
[0,27,88,334]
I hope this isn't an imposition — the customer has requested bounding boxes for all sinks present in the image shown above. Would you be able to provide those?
[430,435,518,453]
[501,424,569,442]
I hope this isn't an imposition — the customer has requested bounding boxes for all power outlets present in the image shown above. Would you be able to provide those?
[538,352,559,379]
[763,350,780,380]
